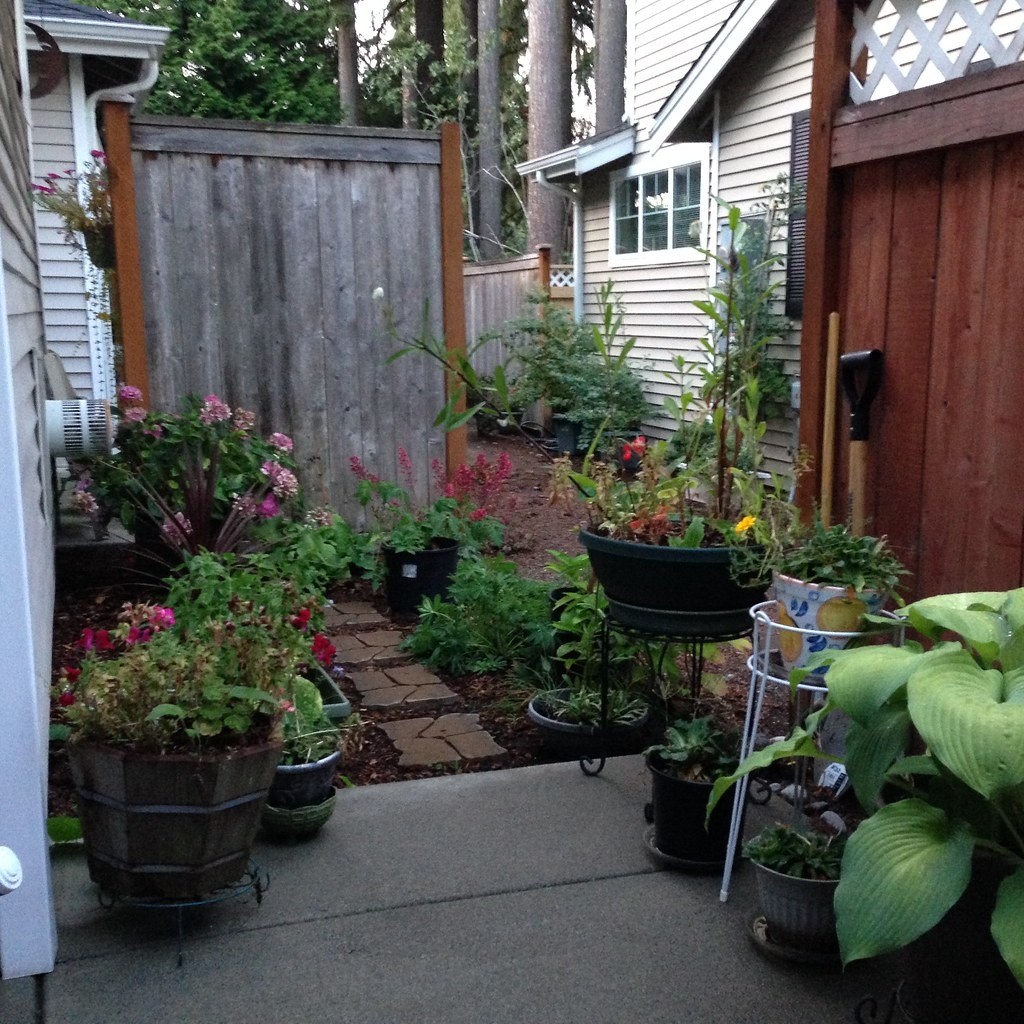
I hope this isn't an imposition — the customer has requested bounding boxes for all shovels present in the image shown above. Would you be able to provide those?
[813,345,882,805]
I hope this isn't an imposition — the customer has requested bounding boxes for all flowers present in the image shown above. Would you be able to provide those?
[31,151,123,406]
[50,383,339,794]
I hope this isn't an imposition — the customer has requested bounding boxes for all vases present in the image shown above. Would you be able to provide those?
[63,663,350,938]
[82,224,115,270]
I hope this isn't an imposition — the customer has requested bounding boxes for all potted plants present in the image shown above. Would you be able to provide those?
[346,171,1023,1024]
